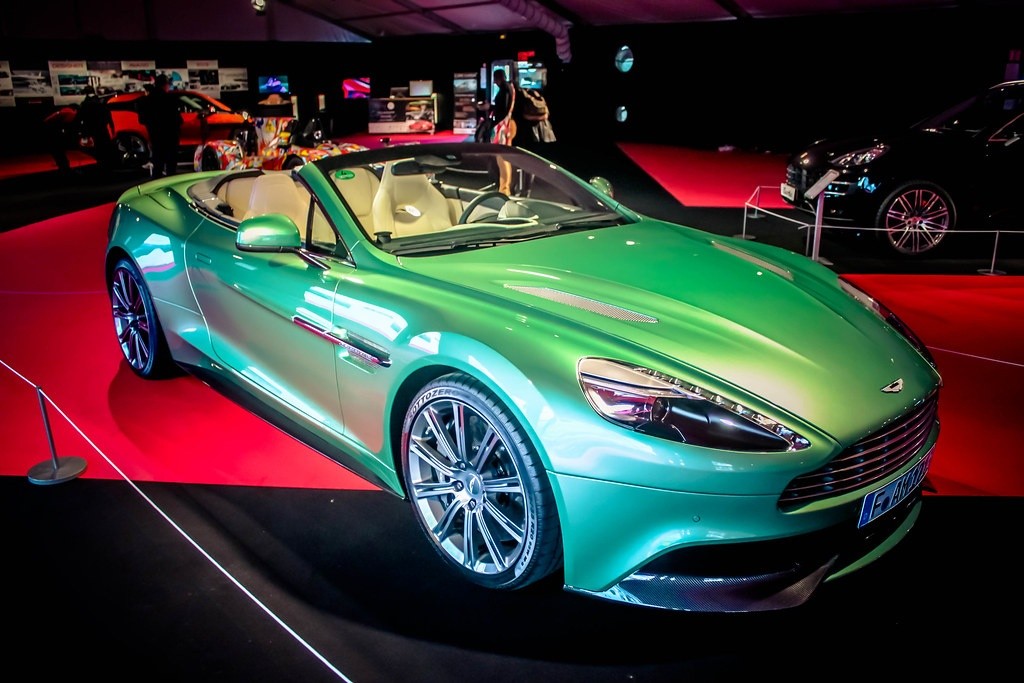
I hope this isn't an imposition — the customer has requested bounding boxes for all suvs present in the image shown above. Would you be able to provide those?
[783,79,1024,260]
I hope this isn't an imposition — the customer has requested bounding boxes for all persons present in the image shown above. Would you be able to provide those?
[265,77,287,93]
[488,69,557,195]
[140,82,157,169]
[136,74,184,181]
[66,85,117,185]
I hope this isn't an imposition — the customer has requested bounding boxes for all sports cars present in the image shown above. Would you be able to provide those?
[102,136,944,619]
[192,127,395,183]
[49,82,262,164]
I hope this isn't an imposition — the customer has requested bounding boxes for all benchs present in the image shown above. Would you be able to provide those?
[222,167,379,241]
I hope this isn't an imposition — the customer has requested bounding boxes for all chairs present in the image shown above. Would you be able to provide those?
[372,157,452,239]
[243,175,336,247]
